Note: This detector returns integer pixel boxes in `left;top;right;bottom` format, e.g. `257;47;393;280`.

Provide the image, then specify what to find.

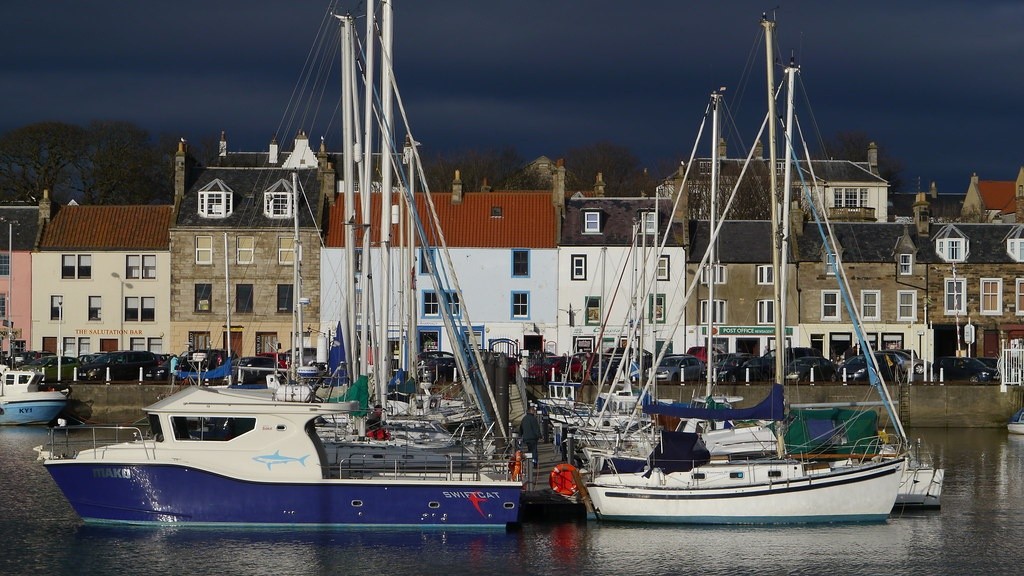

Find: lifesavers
509;456;527;474
549;463;579;497
877;431;889;444
512;451;521;480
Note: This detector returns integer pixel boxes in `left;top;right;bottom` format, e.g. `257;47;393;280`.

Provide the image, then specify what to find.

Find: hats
374;405;381;408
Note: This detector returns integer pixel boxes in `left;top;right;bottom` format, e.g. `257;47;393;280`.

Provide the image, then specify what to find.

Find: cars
14;348;316;385
416;351;458;382
933;356;1022;383
508;346;831;386
831;349;930;382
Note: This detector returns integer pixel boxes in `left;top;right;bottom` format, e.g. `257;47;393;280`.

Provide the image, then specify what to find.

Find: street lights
0;217;20;358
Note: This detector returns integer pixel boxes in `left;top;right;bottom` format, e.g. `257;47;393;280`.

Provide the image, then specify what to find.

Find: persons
585;352;592;368
519;406;543;469
370;405;382;421
560;353;566;373
170;355;179;380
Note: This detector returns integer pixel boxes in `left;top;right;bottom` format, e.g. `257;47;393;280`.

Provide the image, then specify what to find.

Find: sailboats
34;4;540;541
530;5;945;525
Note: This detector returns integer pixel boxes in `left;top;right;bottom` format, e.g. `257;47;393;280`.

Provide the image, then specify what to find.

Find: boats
0;363;69;425
1007;407;1024;434
